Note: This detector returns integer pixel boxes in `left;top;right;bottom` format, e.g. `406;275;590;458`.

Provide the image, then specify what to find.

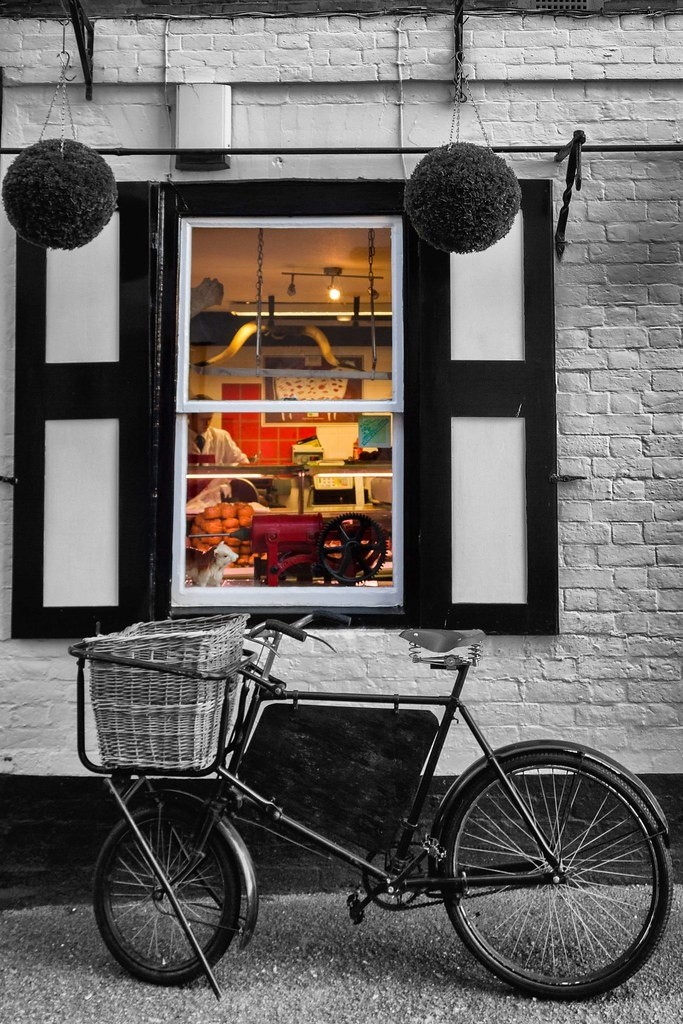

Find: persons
185;393;267;518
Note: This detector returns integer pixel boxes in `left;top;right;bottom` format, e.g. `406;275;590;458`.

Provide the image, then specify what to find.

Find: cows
185;541;239;588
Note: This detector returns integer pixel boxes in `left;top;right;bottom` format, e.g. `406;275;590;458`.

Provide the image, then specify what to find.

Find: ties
195;435;204;451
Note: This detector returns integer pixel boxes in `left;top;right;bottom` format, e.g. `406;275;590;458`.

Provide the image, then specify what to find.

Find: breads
186;500;267;568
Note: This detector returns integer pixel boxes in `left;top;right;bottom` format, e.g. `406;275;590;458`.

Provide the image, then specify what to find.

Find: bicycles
68;610;672;1000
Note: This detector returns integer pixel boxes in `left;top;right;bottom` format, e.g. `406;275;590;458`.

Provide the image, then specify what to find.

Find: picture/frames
259;354;364;427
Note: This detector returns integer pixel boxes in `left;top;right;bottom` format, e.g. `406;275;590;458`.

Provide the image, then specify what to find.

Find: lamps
278;265;384;303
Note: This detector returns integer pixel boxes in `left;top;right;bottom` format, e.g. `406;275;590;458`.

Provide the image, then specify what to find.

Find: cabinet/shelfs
186;460;393;588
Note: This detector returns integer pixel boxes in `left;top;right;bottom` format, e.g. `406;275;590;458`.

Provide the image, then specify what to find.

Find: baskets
84;612;252;770
